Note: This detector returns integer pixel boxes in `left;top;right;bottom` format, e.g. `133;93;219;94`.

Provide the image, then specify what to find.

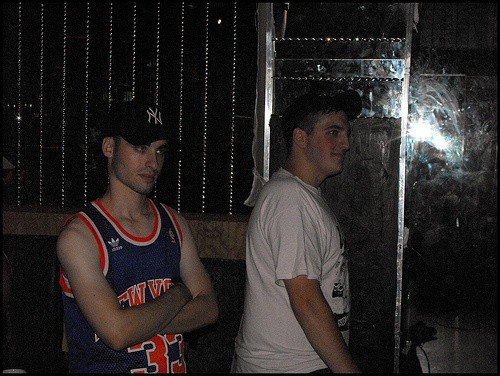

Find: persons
230;90;364;376
57;97;218;376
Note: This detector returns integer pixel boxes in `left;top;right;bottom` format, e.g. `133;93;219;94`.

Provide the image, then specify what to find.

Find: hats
280;88;363;144
103;98;173;146
